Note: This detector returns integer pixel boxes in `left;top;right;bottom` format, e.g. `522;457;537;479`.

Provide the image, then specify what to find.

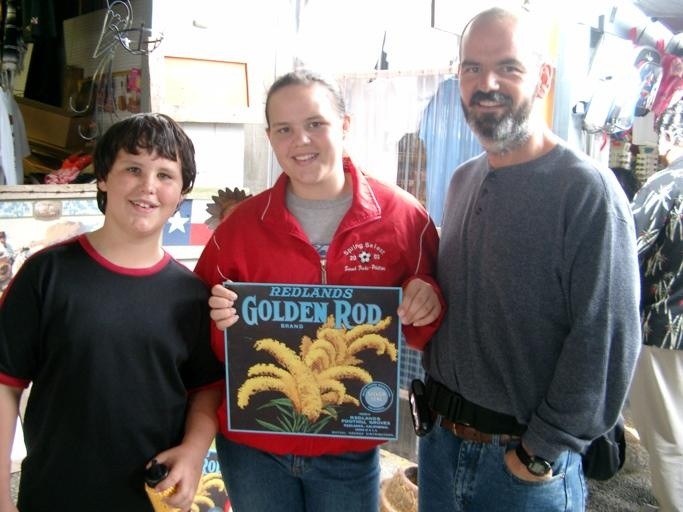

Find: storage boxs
10;95;89;151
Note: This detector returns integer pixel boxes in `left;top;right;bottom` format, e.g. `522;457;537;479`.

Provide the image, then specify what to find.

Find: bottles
143;464;183;512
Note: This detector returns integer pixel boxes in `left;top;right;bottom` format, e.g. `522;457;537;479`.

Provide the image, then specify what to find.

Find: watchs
516;439;552;477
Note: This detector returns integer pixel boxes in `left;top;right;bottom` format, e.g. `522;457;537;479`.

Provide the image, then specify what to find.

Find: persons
407;5;643;512
630;102;683;512
0;114;222;512
611;167;642;201
190;69;446;512
0;231;20;290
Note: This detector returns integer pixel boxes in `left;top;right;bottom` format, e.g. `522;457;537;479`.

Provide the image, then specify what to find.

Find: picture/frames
157;45;263;127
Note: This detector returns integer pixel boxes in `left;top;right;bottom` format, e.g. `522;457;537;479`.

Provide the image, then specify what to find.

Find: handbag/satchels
578;415;626;481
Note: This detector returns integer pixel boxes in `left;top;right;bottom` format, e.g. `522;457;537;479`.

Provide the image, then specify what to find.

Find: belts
440;417;519;446
